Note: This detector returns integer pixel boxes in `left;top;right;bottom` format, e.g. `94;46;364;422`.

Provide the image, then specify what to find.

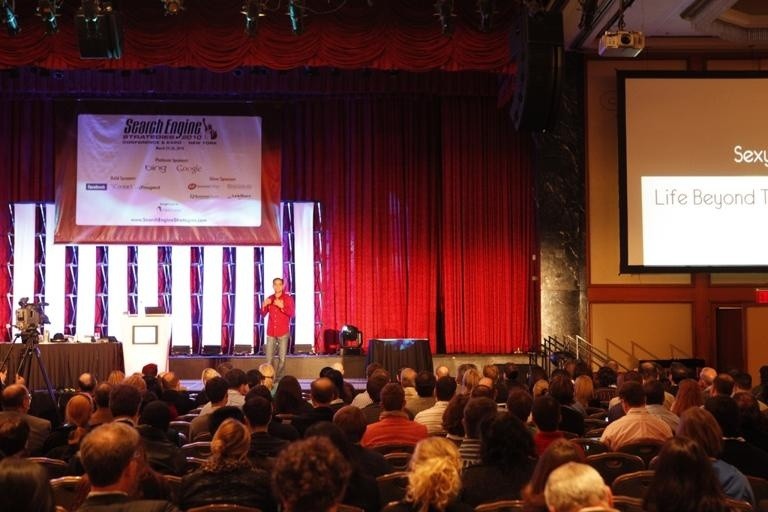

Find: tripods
0;332;58;409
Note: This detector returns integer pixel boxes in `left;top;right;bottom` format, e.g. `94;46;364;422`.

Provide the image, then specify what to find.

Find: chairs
0;376;768;512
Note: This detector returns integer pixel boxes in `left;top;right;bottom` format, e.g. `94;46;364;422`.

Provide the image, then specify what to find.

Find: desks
0;339;125;392
366;338;433;383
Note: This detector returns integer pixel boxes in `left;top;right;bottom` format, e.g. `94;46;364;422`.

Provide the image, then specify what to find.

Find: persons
1;379;53;453
0;361;27;404
0;459;57;511
260;278;295;382
47;361;768;512
0;415;29;456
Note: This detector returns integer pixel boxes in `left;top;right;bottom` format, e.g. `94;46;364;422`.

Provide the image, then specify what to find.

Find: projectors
599;31;645;57
